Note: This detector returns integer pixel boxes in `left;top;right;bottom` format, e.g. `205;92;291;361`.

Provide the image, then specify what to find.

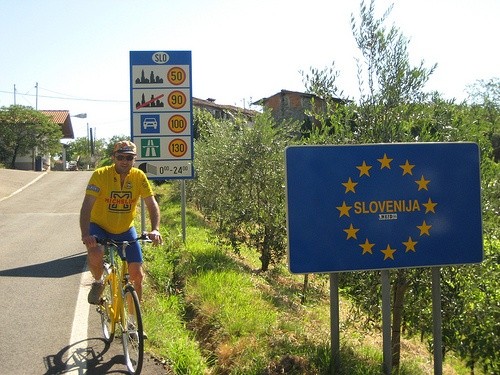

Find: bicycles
83;232;164;375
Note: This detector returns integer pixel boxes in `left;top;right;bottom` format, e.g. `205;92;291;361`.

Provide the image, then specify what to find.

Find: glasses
115;155;133;161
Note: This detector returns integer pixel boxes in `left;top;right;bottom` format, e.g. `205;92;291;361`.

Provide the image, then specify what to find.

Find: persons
79;141;164;339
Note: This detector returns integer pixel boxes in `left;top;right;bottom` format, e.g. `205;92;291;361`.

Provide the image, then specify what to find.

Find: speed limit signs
168;114;187;133
167;66;186;85
169;137;188;157
167;89;187;110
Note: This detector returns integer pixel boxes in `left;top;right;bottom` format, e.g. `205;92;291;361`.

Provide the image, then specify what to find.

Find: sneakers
128;321;148;340
88;275;107;305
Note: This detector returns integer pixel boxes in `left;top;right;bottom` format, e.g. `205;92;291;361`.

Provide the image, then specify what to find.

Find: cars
66;160;79;171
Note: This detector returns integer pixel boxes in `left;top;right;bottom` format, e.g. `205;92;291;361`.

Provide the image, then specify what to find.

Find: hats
112;141;137;155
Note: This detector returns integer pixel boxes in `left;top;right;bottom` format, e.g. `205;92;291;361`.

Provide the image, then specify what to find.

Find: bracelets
151;228;158;231
